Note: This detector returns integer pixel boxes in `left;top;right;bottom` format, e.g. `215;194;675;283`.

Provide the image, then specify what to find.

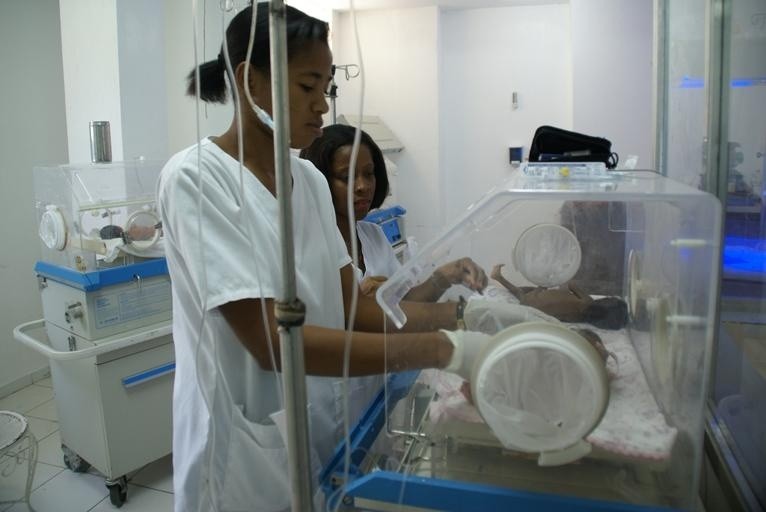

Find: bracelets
431;273;446;292
456;296;466;331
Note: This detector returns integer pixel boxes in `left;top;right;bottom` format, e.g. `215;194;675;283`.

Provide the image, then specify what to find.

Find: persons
459;329;607;404
300;124;489;427
490;263;629;331
100;223;163;240
158;2;561;512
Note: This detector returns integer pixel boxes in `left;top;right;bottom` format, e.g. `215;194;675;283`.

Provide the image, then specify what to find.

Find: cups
88;120;112;163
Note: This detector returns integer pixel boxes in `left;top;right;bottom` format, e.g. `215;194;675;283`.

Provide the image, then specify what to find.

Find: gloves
463;295;559;333
437;328;492;380
